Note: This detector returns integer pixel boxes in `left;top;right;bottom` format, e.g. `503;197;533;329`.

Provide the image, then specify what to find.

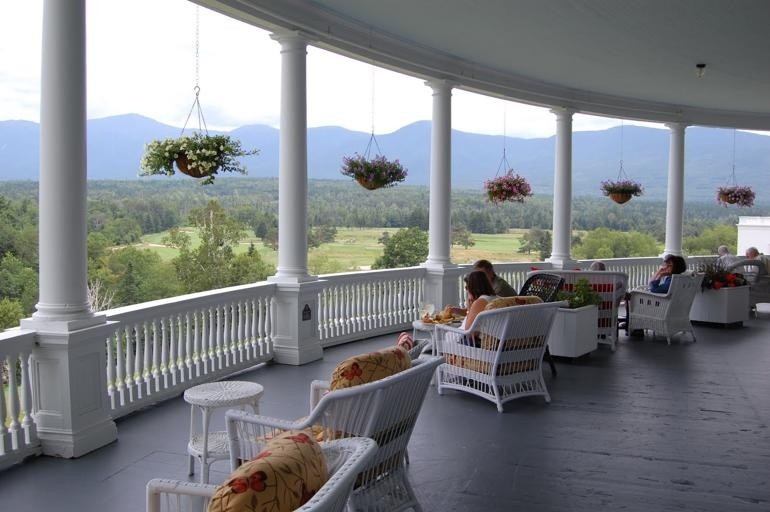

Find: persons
618;253;685;338
715;245;744;277
473;258;519;296
746;247;764;274
448;271;502;365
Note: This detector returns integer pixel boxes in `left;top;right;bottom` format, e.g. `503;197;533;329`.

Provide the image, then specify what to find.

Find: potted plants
689;262;751;328
539;277;604;367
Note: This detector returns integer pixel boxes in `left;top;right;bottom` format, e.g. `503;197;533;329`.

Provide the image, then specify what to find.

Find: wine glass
419;303;434;321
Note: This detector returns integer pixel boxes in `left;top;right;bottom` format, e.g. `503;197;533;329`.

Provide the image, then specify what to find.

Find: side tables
411;317;464;386
183;380;265;484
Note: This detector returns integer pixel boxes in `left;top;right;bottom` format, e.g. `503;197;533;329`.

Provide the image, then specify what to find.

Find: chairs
526;270;629;352
144;438;378;512
436;298;571;413
225;355;445;512
628;269;706;345
727;258;770;321
518;272;565;376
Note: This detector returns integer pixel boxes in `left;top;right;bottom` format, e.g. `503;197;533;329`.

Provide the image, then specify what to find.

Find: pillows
329;347;411;493
207;430;329;512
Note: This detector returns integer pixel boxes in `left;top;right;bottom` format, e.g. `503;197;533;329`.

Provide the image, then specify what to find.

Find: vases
721;193;746;204
493;189;514;198
356;175;387;190
609;191;634;204
174;153;220;178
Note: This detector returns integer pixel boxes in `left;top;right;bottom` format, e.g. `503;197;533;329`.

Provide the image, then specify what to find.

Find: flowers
599;177;646;198
713;184;756;209
136;132;261;185
339;151;409;189
483;166;535;206
